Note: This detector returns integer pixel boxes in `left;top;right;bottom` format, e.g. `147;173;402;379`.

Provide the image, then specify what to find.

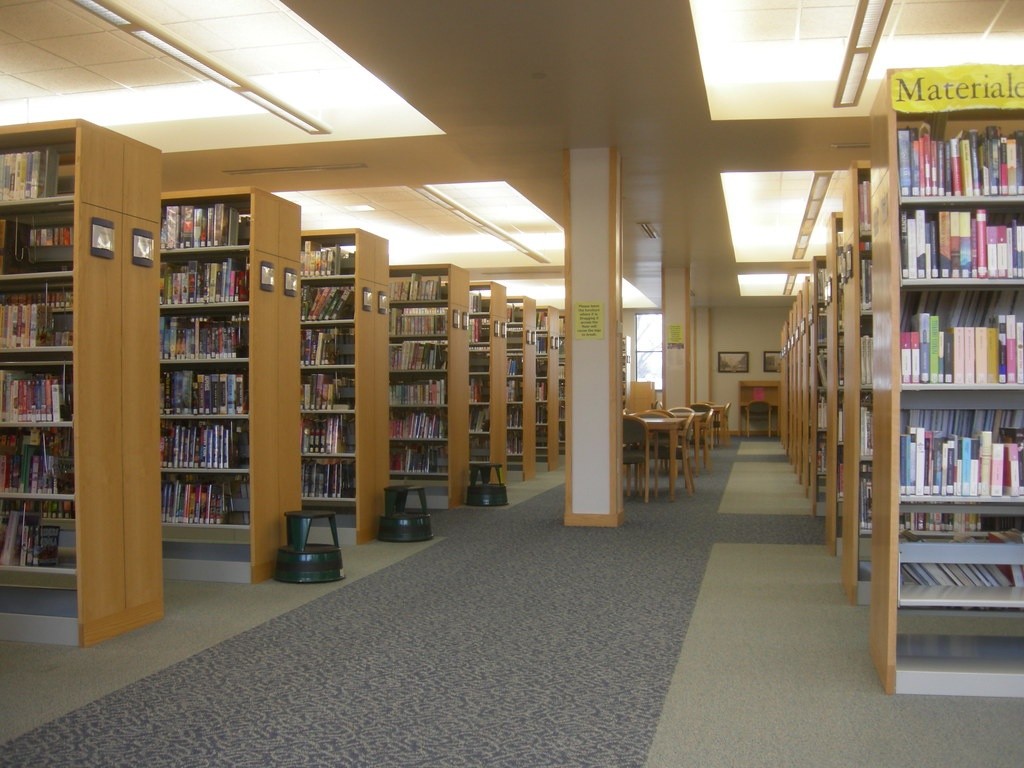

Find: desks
739;380;781;438
639;418;689;500
673;411;708;479
710;404;726;447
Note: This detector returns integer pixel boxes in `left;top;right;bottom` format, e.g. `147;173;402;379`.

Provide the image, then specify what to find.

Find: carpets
0;434;829;768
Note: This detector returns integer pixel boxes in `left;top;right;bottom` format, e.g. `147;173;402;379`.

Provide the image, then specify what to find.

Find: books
836;232;844;511
508;306;523;459
859;182;875;537
301;238;354;501
1;150;74;580
534;310;548;460
557;337;567;457
468;293;490;461
161;204;249;528
897;128;1024;607
389;271;448;480
816;269;827;473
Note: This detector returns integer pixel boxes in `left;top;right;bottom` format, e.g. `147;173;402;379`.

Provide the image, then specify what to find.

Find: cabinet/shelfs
630;382;656;410
506;295;538;482
717;351;749;374
389;263;472;511
536;305;560;471
299;228;390;545
779;69;1024;696
160;188;306;589
557;308;564;456
468;281;509;488
623;334;631;412
1;119;163;650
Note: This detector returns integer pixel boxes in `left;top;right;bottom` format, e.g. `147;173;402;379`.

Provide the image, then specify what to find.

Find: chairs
745;400;772;439
623;400;732;503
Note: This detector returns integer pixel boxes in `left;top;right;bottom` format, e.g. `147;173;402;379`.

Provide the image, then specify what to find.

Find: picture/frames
763;350;782;373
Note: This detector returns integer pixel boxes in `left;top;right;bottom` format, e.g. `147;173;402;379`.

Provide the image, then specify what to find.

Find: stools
273;508;342;584
377;484;433;542
465;460;509;507
